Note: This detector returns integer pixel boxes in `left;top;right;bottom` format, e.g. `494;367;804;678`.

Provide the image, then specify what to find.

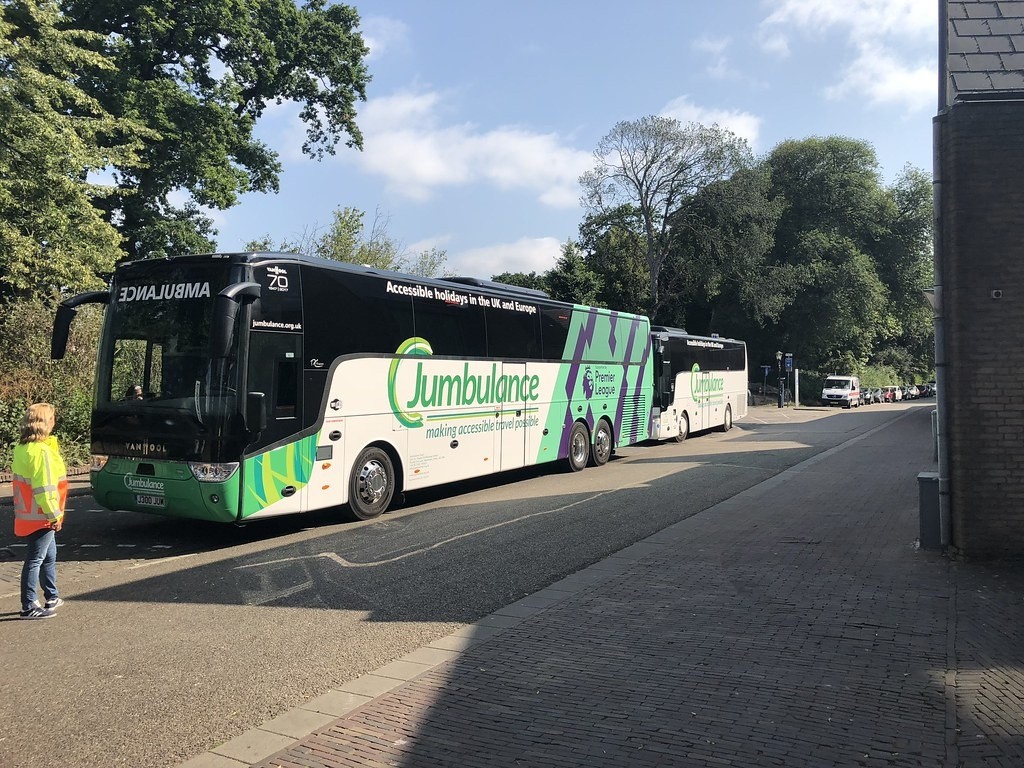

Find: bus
51;248;654;526
650;326;749;443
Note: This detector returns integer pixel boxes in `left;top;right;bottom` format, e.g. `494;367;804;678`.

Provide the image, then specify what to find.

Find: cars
885;386;903;401
907;386;920;399
880;387;895;402
860;387;874;405
926;385;934;396
870;388;884;403
899;386;912;400
916;385;929;397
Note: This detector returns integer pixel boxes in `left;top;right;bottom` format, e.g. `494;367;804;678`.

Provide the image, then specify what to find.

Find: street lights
775;351;783;408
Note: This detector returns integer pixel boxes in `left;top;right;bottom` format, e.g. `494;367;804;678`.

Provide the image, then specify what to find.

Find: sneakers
45;598;64;612
19;602;58;619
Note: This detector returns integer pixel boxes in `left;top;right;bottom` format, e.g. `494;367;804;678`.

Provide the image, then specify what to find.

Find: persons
13;403;65;619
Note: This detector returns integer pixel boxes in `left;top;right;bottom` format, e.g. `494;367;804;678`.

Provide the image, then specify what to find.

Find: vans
821;376;859;407
